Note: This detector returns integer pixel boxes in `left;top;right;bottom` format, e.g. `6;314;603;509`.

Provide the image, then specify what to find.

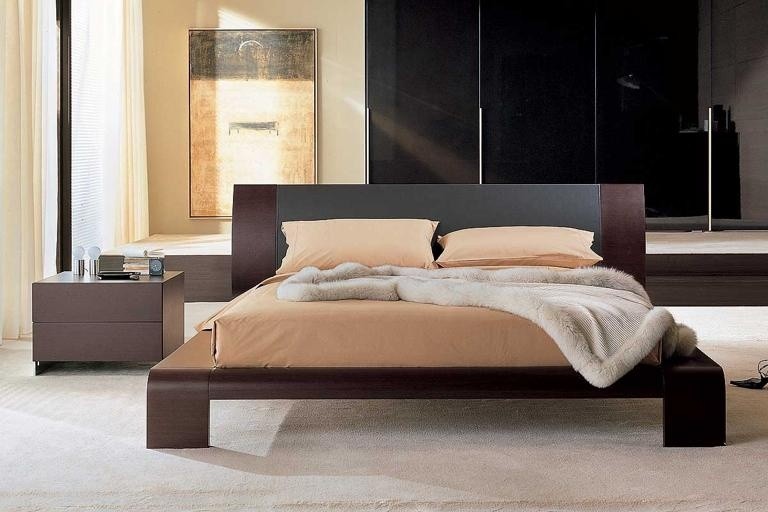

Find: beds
146;184;725;449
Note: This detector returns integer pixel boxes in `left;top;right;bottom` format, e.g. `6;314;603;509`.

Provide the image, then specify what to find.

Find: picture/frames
188;27;318;220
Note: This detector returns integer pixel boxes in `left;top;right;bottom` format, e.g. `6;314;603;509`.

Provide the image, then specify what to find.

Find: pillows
433;225;603;270
275;219;441;275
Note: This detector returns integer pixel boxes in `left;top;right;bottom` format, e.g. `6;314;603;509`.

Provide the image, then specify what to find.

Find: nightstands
32;269;184;376
